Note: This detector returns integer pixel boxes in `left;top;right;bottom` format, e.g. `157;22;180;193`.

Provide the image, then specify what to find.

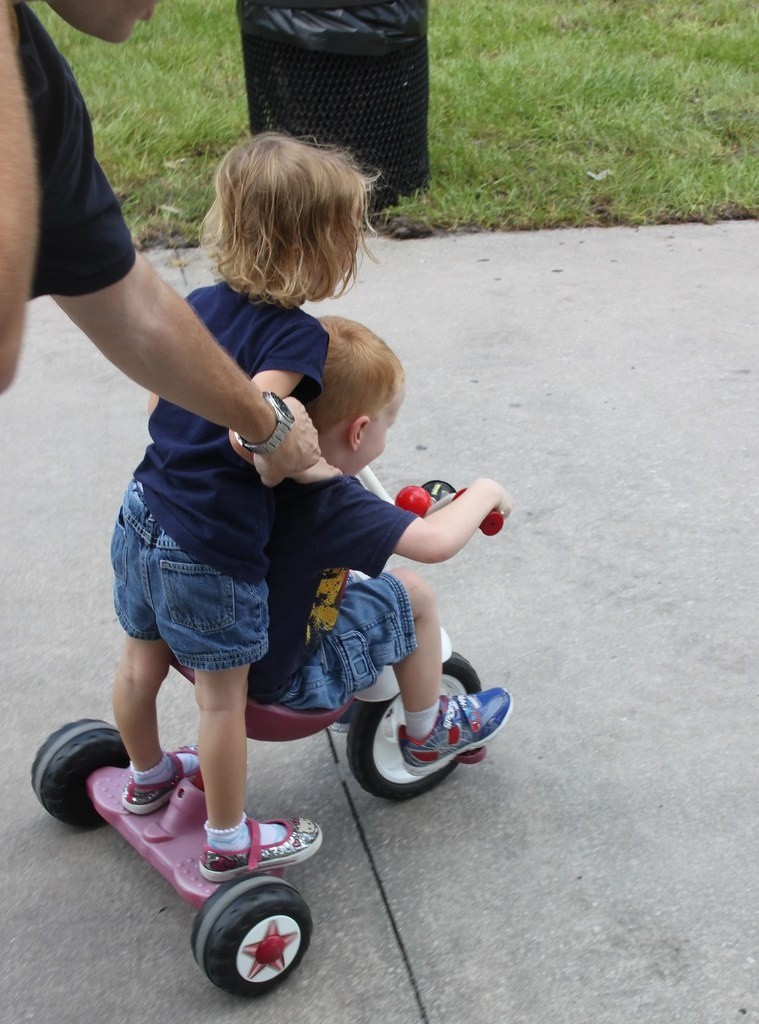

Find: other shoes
199;818;322;882
122;743;201;815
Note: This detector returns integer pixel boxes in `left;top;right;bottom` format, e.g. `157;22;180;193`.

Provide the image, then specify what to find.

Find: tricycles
34;480;503;998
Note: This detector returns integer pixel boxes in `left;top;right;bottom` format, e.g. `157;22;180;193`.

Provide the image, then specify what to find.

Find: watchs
232;391;295;457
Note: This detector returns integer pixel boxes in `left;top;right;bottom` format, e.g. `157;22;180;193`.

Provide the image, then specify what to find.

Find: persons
109;122;515;884
0;0;323;487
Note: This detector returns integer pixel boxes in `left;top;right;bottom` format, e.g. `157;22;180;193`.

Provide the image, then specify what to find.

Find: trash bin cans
234;0;430;222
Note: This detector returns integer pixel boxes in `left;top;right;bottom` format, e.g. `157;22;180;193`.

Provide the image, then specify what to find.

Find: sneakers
398;687;512;776
